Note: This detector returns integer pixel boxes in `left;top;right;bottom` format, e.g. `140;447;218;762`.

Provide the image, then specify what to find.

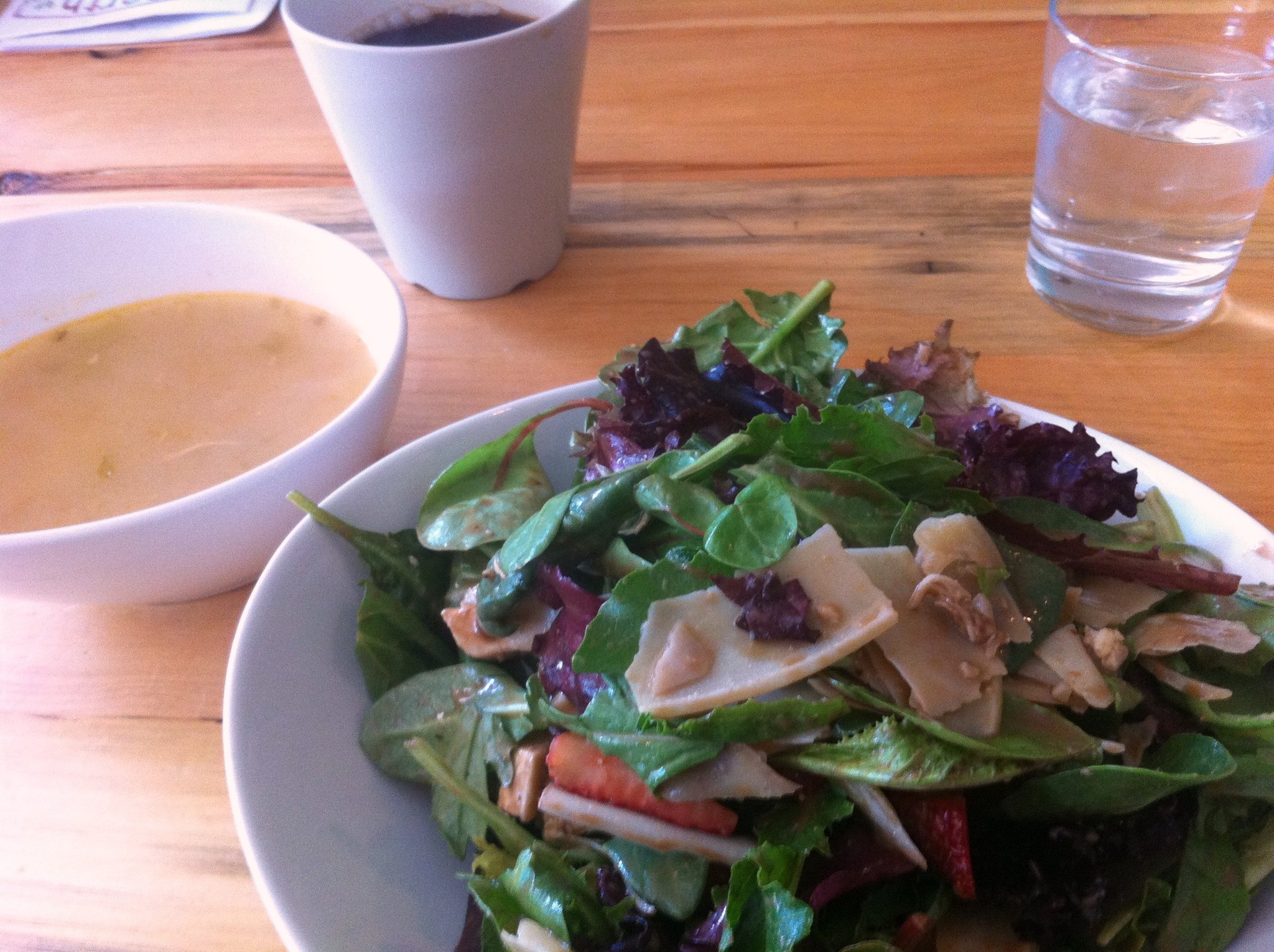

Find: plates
220;379;1274;952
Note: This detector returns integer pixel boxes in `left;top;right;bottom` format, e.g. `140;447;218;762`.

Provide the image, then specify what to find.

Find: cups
1026;0;1273;339
279;1;592;300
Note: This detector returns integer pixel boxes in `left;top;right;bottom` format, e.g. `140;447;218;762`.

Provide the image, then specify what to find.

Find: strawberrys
901;784;975;898
545;731;738;836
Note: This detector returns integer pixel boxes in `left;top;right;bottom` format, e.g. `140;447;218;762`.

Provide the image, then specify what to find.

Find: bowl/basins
0;203;406;605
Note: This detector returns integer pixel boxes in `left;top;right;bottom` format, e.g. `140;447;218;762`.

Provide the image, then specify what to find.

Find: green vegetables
285;278;1273;952
